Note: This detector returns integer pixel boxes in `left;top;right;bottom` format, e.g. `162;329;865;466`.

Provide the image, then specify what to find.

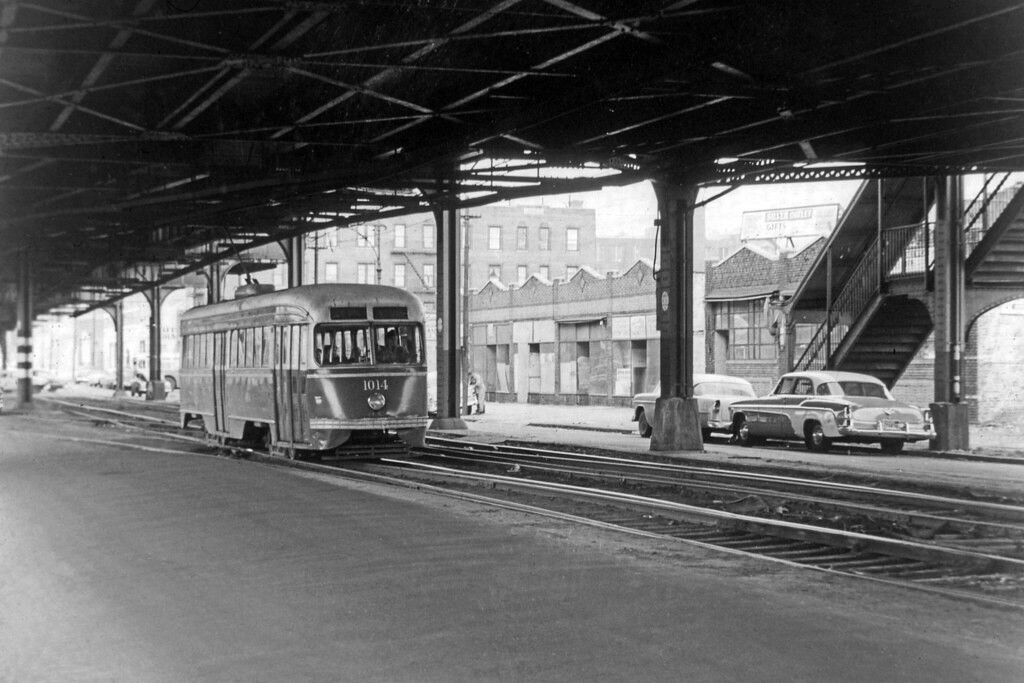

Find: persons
468;371;486;414
379;330;411;364
314;348;321;365
349;347;362;363
332;346;340;364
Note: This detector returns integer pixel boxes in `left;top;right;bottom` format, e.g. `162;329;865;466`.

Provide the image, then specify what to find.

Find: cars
728;371;937;455
163;364;181;391
632;374;758;441
128;371;172;398
74;366;133;390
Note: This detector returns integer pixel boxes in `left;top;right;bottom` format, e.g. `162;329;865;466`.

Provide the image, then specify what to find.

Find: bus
179;283;447;460
